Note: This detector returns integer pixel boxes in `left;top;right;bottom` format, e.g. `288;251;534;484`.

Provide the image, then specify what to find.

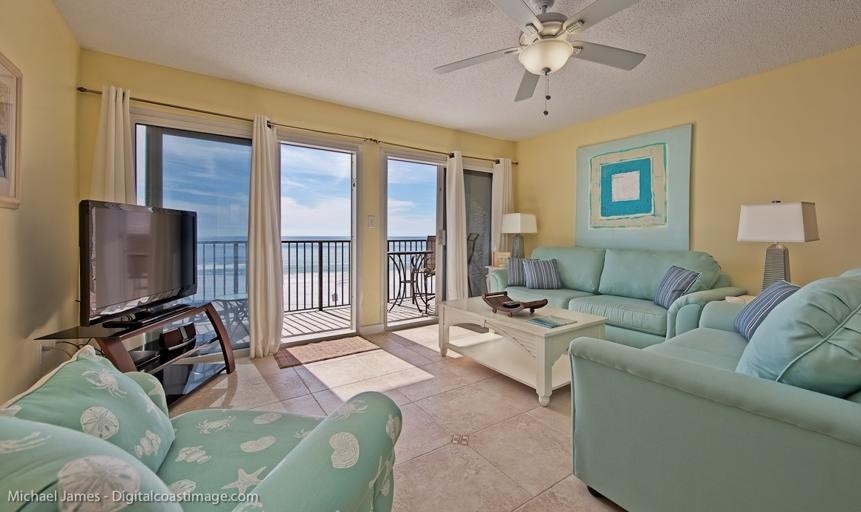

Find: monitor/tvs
78;198;197;327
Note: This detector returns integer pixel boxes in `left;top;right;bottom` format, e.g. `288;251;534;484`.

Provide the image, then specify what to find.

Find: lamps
735;200;821;295
500;210;539;257
515;38;575;115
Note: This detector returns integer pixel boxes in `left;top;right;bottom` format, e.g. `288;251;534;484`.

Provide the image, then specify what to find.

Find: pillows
507;256;563;288
4;341;179;471
655;263;704;308
735;269;861;398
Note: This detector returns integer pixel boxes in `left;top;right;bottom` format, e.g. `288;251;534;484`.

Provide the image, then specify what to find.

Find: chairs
0;368;409;509
564;269;861;512
413;234;437;311
465;230;480;296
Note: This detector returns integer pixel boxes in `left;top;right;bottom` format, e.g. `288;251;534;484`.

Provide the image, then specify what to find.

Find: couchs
487;244;732;349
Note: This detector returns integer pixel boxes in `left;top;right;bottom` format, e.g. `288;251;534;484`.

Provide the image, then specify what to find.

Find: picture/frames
1;53;24;212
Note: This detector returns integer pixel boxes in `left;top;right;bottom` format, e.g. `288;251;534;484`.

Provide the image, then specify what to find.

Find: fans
434;1;650;103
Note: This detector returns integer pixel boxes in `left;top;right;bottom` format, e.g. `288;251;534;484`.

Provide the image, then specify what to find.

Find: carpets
273;335;384;368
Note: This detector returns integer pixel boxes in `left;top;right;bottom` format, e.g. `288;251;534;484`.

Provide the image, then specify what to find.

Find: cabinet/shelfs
32;297;239;409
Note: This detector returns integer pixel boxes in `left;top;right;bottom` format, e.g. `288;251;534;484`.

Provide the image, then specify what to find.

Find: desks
386;250;435;315
216;293;250;344
